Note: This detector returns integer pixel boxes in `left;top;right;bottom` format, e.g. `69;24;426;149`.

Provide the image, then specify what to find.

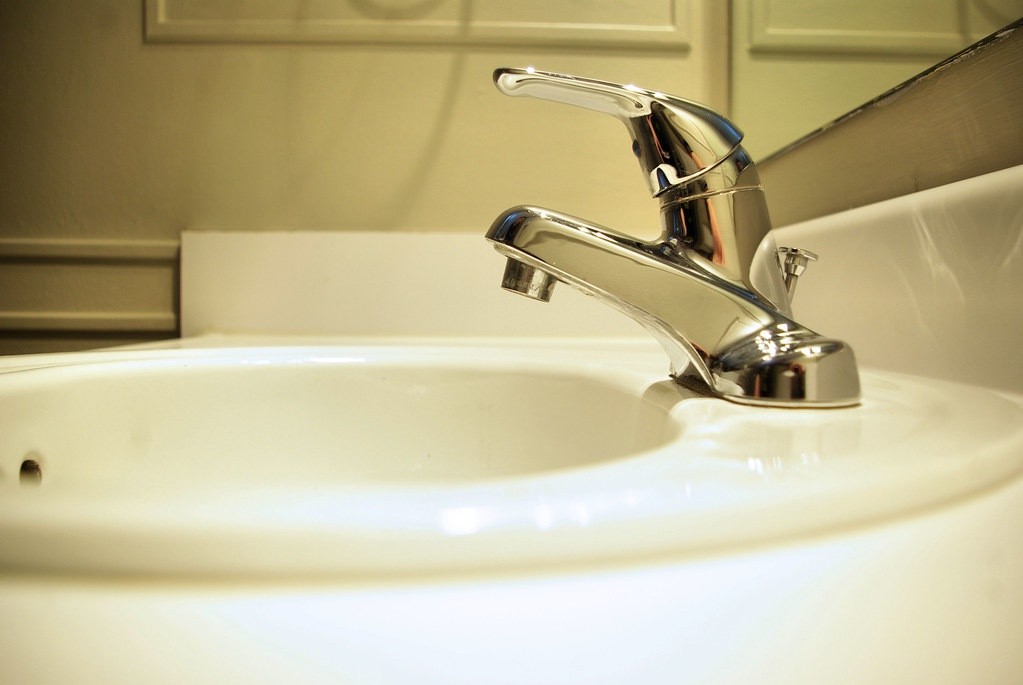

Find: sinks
0;339;1022;663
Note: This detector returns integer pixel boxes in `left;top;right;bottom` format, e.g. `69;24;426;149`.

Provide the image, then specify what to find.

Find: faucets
483;66;863;411
